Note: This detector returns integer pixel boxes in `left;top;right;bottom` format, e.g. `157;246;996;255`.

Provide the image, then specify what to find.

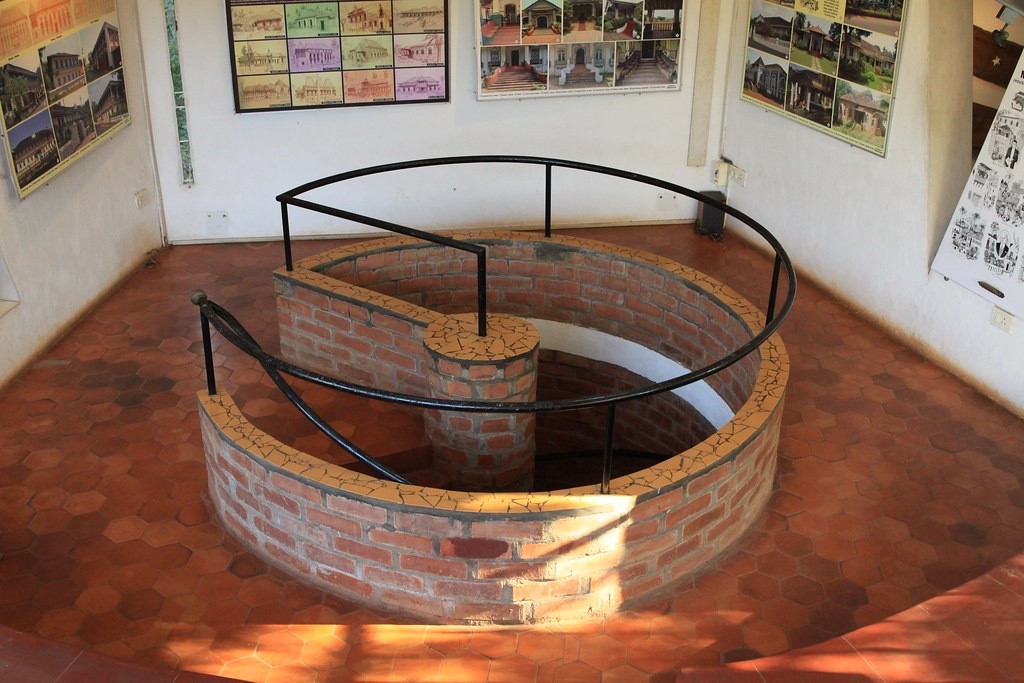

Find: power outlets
134;189;149;210
728;164;746;187
